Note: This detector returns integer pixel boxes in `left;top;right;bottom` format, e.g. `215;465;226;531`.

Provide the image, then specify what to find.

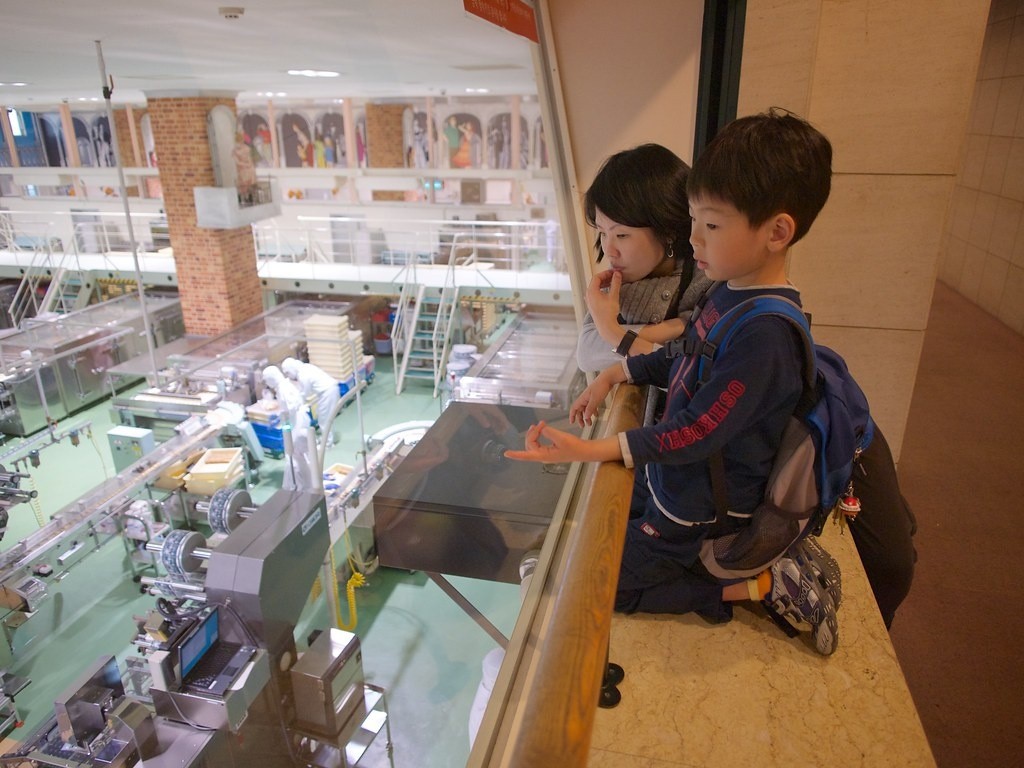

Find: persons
576;141;918;634
501;105;866;659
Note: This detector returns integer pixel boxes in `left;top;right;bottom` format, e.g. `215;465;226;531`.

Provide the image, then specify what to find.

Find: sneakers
765;539;841;660
783;529;841;615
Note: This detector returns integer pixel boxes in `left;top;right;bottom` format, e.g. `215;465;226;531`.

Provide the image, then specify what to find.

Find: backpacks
663;274;876;583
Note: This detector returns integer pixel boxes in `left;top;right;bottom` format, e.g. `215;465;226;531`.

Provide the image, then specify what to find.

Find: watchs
608;328;639;361
